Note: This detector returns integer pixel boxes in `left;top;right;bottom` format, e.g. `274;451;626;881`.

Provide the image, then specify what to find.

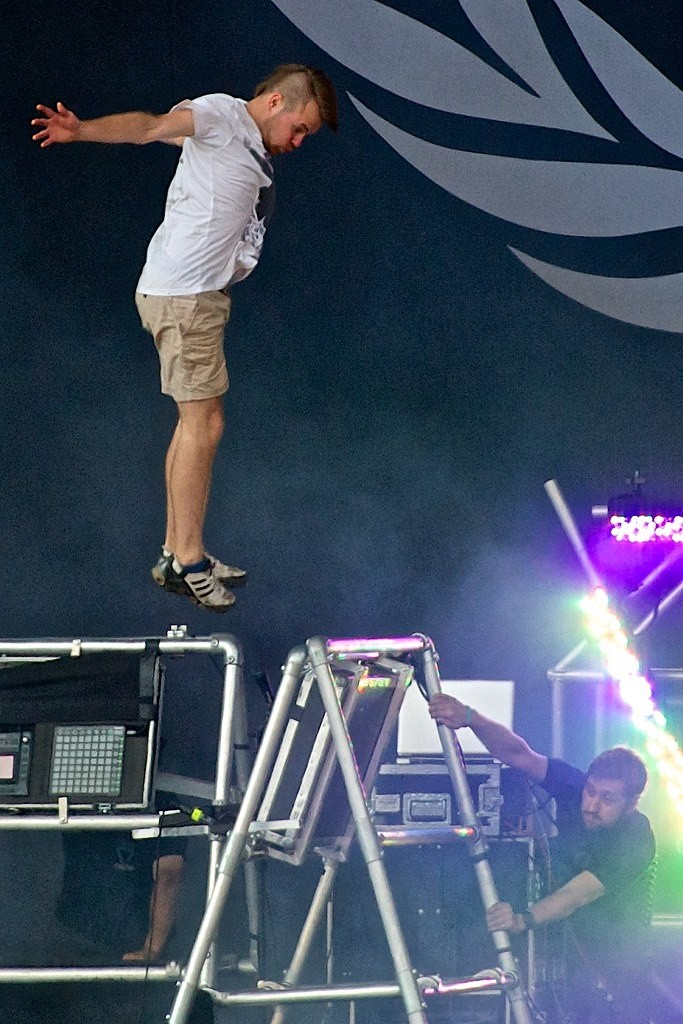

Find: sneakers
156;547;248;591
152;554;236;613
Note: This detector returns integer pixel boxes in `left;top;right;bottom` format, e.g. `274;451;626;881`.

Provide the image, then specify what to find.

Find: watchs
518;910;532;934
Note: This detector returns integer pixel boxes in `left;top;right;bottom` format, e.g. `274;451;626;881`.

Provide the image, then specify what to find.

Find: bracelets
465;705;471;728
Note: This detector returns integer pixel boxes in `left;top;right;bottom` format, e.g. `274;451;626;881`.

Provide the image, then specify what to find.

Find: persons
429;689;659;1024
48;772;192;1024
29;63;334;613
